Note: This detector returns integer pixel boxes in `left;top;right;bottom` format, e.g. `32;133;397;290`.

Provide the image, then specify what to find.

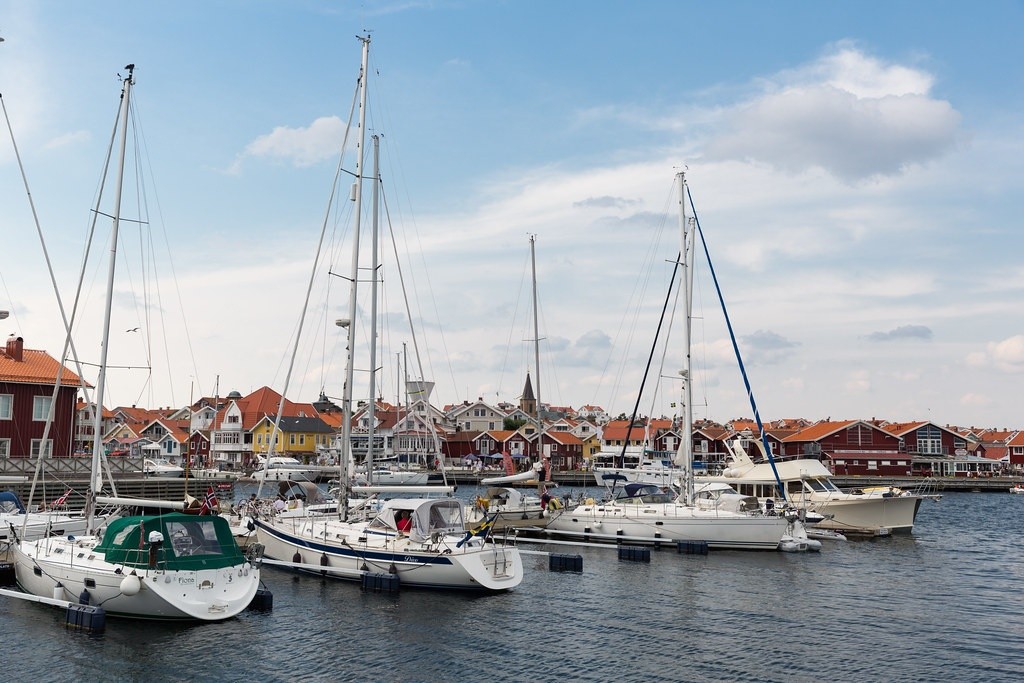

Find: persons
289;495;299;511
396;511;412;531
921;466;926;476
247;493;263;518
270;496;284;517
169;458;178;465
434;458;438;471
244;456;248;468
229;503;242;519
161;456;168;464
465;459;477;470
928;467;932;477
888;485;911;497
181;457;187;464
533;465;560;510
254;457;259;468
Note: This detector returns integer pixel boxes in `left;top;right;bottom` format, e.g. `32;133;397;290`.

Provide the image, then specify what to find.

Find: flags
198;486;219;517
50;487;73;509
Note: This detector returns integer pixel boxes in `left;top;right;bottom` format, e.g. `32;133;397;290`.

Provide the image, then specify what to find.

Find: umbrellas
464;453;478;460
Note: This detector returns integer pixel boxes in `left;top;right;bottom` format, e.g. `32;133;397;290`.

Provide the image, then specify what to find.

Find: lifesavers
548;499;560;512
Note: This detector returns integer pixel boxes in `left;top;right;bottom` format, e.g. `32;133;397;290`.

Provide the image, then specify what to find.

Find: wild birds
126;327;141;333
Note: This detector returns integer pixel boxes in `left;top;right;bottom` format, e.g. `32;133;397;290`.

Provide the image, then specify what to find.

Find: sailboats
0;34;925;622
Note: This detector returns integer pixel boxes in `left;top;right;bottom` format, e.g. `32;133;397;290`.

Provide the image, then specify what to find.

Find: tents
477;452;526;472
